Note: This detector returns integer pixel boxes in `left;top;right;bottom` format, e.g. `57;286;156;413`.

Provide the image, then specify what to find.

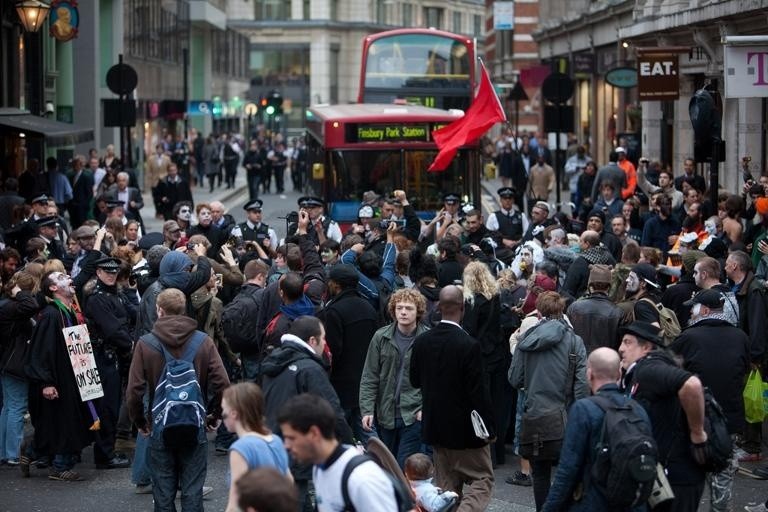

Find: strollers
355;434;464;512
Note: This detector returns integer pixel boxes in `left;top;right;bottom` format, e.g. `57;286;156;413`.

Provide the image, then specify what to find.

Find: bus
357;26;485;120
305;100;486;227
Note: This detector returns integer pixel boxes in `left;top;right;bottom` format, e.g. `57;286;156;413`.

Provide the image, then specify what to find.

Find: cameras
747;179;757;187
129;267;142;287
286;211;304;221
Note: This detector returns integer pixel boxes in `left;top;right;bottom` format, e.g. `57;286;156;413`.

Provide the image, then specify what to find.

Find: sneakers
731;432;768;511
134;481;153;496
0;450;81;481
505;471;532;488
175;483;213;500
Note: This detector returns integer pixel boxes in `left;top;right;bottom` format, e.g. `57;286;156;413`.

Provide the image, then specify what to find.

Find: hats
682;288;725;308
535;204;548;211
103;200;125;213
443;194;462;205
326;265;359;288
587;208;605;225
497;187;516;198
587;263;613;297
632;262;659;288
77;224;95;238
29;189;60;226
620;322;661;345
297;195;326;208
407;250;438;281
521;274;555;314
139;220;181;252
243;198;263;212
616;146;627;153
92;255;123;274
358;188;407;236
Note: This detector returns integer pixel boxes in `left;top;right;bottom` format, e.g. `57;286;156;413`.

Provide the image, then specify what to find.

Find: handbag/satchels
470;410;489;439
517;409;568;463
632;299;681;347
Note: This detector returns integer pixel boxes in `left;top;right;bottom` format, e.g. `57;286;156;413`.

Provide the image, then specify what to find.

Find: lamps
11;0;50;41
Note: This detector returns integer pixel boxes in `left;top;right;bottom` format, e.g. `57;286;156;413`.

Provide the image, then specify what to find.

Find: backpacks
220;287;260;355
378;281;393;327
633;356;734;475
140;332;209;461
584;395;659;512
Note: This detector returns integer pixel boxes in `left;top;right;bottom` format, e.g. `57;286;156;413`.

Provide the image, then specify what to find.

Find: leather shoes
95;439;136;470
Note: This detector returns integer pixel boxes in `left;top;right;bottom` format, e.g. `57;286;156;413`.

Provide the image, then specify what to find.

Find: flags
427;66;505;171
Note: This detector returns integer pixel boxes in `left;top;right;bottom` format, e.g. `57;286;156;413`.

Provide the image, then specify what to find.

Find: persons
0;125;766;512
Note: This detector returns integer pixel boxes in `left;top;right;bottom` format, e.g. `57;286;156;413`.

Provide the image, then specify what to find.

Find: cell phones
642;160;650;169
187;243;198;251
743;157;751;161
603;206;608;212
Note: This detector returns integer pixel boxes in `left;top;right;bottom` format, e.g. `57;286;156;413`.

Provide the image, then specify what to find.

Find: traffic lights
257;98;275;120
274;95;287;115
